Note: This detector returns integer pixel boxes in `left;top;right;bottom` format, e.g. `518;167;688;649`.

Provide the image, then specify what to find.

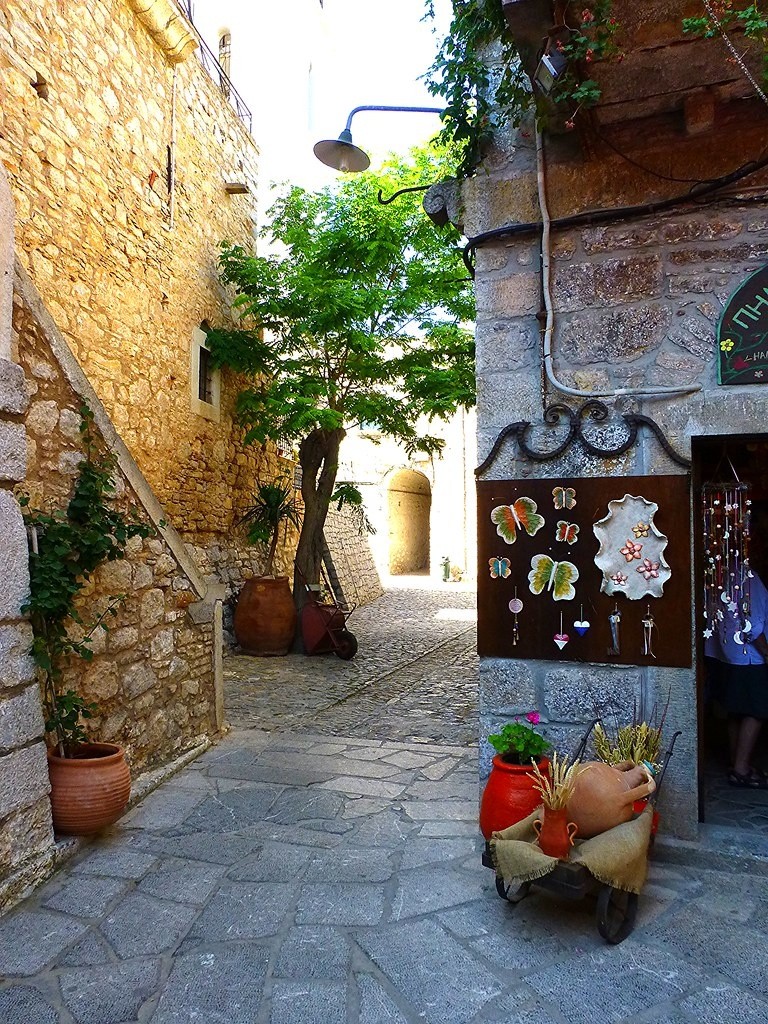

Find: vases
532;803;579;859
480;756;555;842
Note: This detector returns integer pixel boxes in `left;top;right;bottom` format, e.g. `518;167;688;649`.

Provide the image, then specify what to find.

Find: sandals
727;764;764;777
729;769;768;789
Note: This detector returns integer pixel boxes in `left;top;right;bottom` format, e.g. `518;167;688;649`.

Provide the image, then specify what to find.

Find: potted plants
11;397;168;832
232;475;303;656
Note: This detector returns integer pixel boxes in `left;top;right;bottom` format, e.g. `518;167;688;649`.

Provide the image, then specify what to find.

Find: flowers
488;710;549;766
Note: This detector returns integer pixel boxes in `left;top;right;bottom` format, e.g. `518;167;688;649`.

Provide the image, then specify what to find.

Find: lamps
311;106;447;175
532;38;566;97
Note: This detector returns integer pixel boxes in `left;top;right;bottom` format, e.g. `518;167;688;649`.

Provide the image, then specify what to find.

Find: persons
702;529;768;785
